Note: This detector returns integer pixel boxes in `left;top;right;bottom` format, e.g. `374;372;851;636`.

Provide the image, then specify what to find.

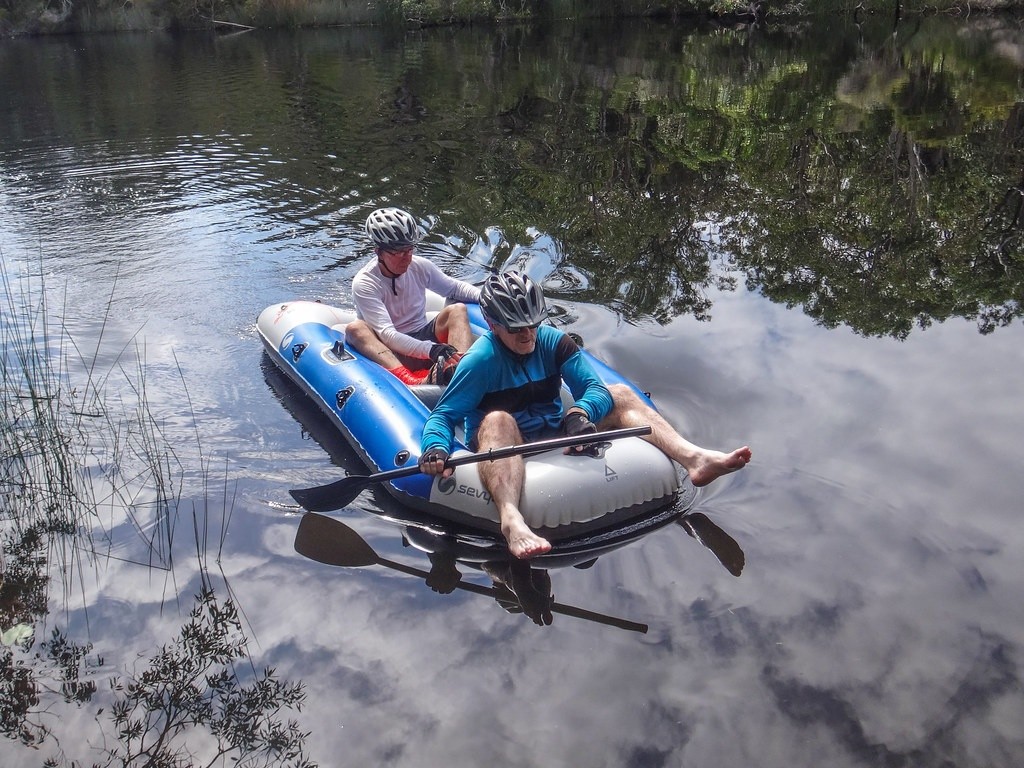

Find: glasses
497;321;541;334
381;246;418;258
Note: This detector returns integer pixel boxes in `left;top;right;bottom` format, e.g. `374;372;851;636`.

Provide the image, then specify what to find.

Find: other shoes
443;364;457;386
417;355;446;385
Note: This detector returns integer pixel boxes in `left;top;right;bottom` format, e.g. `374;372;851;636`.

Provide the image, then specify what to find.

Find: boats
251;298;682;547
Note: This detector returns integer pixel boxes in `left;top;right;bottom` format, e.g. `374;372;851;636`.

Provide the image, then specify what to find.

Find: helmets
365;206;419;250
479;269;549;328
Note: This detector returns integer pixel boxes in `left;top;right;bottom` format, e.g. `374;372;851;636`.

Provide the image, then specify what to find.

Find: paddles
287;426;654;512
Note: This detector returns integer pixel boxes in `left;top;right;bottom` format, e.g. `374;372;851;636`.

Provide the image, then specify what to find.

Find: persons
344;207;484;386
397;511;745;627
420;271;752;561
